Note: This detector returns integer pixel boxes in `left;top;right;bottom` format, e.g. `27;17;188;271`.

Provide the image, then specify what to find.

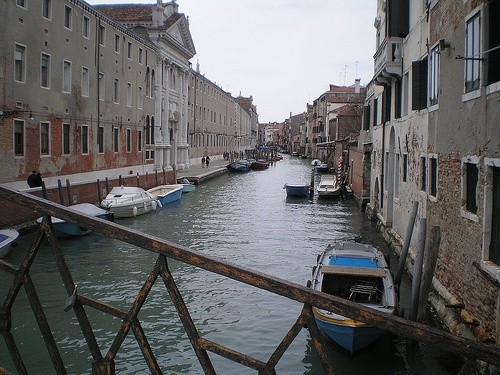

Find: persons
202;156;205;168
206;156;209;168
223;152;229;161
27;170;43;187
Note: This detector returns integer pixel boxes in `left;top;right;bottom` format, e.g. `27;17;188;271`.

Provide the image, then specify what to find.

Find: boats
100;187;157;218
316;174;341;197
228;158;269;173
311;158;328;173
282;183;311;197
268;149;307;161
37;202;115;237
307;234;396;358
145;176;196;206
0;228;20;259
